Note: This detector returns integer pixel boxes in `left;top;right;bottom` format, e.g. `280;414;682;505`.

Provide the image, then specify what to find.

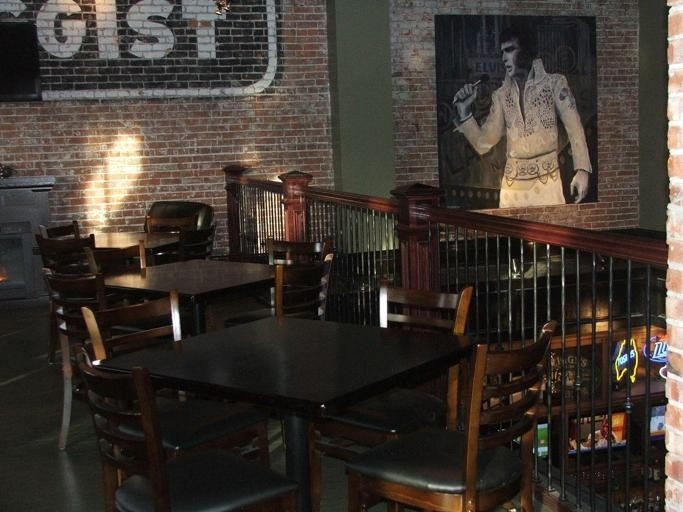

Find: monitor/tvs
568;409;628;454
0;19;43;102
513;423;551;461
648;402;667;444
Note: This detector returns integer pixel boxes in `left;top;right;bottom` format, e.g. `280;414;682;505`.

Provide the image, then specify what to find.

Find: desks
2;175;57;310
37;200;347;418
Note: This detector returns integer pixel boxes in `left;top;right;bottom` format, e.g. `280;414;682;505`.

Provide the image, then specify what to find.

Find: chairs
346;319;552;510
311;281;476;510
76;348;272;511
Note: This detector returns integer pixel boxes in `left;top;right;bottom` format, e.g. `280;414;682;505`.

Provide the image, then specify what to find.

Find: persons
448;16;594;211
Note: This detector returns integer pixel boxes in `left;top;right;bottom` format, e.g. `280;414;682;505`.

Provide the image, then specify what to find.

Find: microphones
452;75;489;103
